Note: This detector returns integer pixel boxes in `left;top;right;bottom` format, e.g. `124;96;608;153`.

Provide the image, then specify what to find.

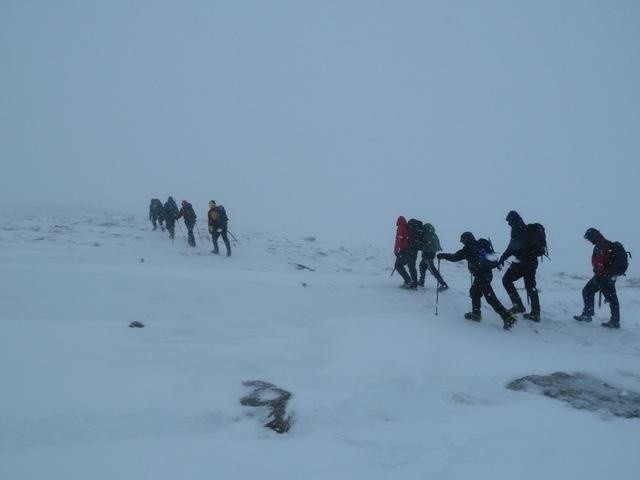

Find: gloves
497;257;504;271
437;253;447;259
395;252;399;256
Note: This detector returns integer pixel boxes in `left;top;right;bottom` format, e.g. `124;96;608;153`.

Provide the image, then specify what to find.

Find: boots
523;305;540;322
464;310;481;322
508;299;526;314
501;312;516;329
438;282;448;291
417;278;424;287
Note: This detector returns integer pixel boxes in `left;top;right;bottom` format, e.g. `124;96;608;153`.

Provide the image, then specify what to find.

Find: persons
149;198;165;232
572;227;621;328
393;215;418;289
416;223;448;291
497;210;541;322
437;232;515;329
176;200;197;247
207;200;231;257
160;197;179;239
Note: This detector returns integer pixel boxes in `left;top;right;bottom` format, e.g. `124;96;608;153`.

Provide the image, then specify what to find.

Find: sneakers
212;249;219;255
573;313;592;322
227;249;231;256
402;280;417;289
601;321;620;328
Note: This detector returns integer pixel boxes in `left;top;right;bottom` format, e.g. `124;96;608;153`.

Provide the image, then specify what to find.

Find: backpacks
215;205;228;224
607;242;628;275
525;223;546;258
473;238;499;271
422;223;440;252
407;219;423;250
